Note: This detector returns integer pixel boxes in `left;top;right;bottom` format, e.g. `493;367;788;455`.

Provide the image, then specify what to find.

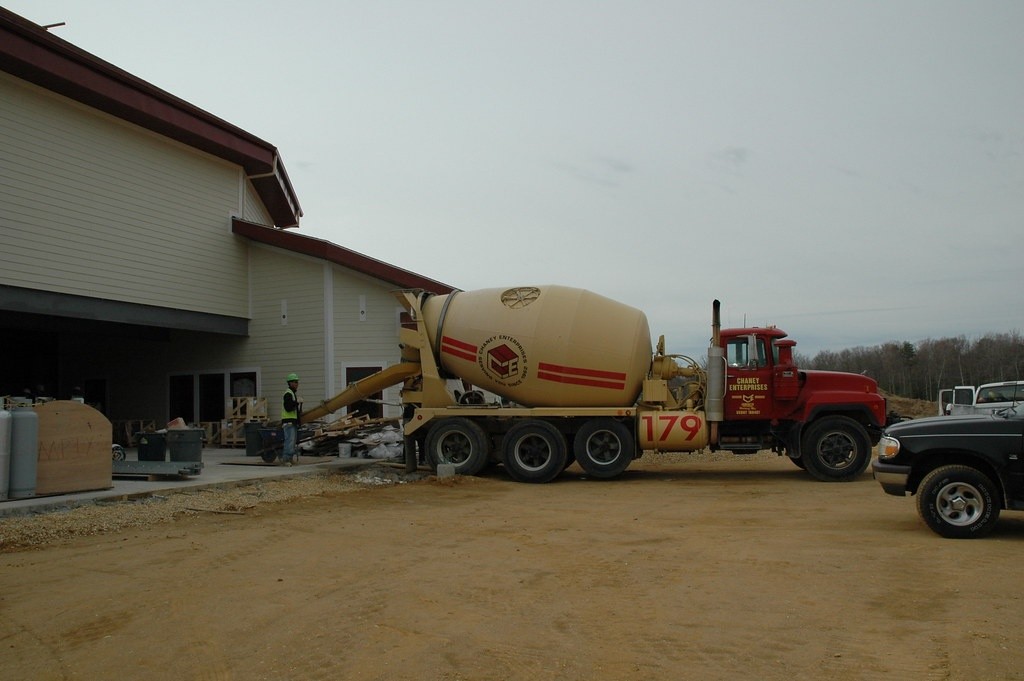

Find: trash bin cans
243;422;264;457
135;430;168;462
168;428;206;476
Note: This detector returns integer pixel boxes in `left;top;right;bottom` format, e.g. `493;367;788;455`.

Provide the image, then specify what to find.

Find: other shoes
283;460;295;467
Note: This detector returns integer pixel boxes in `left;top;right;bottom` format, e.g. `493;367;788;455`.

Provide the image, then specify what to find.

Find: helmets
286;373;299;381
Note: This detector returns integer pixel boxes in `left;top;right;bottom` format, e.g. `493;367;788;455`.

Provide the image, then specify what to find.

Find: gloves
297;396;303;403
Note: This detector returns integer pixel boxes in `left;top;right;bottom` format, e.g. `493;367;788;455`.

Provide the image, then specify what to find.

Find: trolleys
256;427;328;462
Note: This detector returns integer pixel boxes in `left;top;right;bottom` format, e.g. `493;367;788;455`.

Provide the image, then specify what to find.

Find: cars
872;381;1023;539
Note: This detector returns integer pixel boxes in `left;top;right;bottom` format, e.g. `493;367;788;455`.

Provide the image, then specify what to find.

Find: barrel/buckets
338;443;352;458
244;423;264;456
134;432;166;461
167;428;204;474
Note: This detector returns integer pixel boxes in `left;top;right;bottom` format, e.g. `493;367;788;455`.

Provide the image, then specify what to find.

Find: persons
281;373;303;467
989;391;1003;401
403;405;426;466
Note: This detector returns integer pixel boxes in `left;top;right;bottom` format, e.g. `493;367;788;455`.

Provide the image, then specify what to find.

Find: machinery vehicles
299;283;887;484
